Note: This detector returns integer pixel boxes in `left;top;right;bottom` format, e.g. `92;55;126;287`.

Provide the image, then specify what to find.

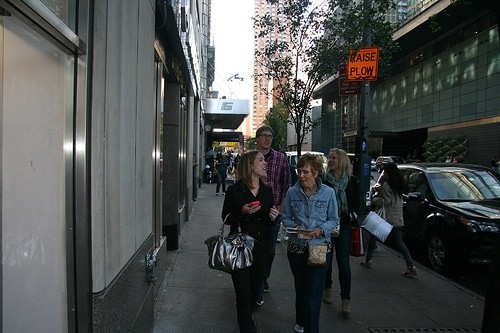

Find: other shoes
257;298;265;305
264;286;271;292
295;325;304;333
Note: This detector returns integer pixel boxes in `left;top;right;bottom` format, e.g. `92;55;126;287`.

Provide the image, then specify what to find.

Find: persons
320;149;362;316
280;154;339;333
222;151;281;333
444;154;461;164
360;162;420;278
490;158;500;175
273;150;297;244
247;125;291;307
203;146;243;196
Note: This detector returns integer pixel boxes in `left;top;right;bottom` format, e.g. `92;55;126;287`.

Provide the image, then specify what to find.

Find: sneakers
360;257;375;267
277;232;283;242
324;288;333;303
284;230;290;241
402;265;417;277
341;300;351;314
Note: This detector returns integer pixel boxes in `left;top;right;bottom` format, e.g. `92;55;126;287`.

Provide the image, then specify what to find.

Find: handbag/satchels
308;243;328;266
362;203;394;242
204;213;257;272
349;212;363;257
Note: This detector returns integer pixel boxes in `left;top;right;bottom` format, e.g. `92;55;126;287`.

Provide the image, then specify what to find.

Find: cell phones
250;201;260;208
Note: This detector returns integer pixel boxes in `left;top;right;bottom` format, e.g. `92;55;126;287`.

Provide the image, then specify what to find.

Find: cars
375;162;500;275
281;148;421;216
